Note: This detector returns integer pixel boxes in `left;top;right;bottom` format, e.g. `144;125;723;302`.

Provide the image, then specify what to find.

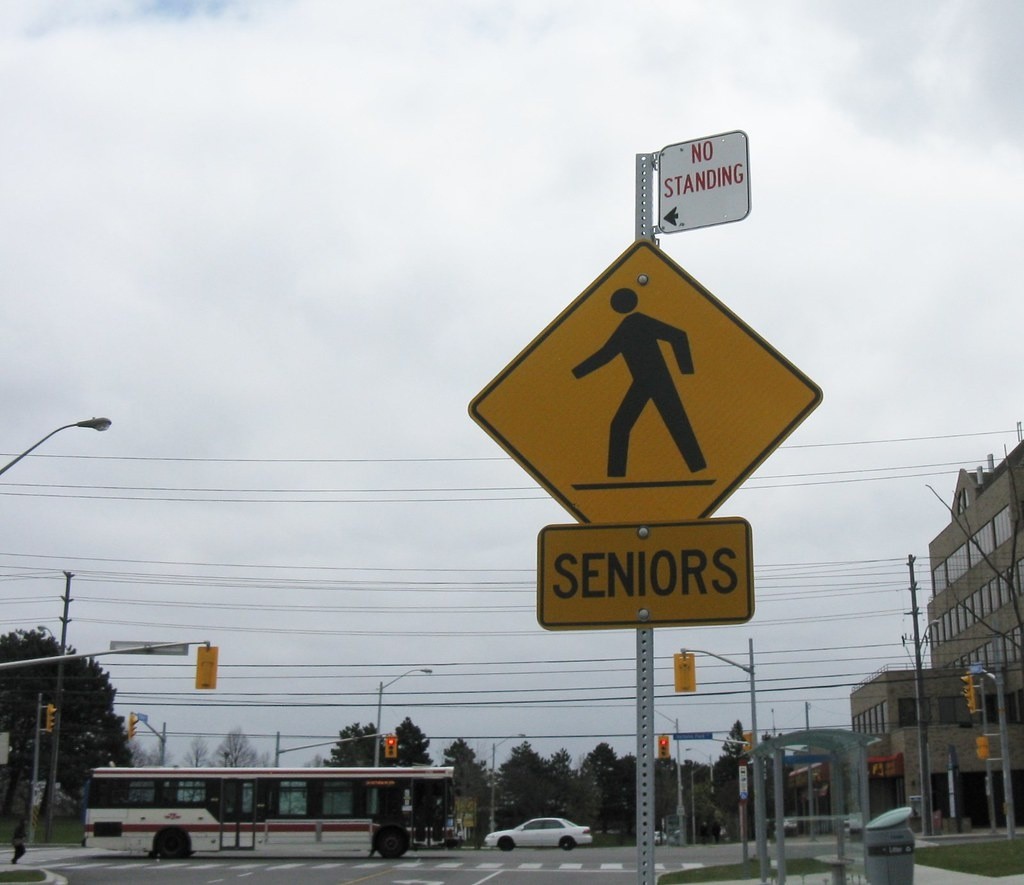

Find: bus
81;767;465;859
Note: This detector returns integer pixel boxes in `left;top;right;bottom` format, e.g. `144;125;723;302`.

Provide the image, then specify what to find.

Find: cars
484;818;592;851
782;819;797;828
645;831;667;844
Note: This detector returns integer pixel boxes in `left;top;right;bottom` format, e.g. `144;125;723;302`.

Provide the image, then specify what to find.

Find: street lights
489;733;526;850
686;747;714;793
913;621;942;837
375;669;432;768
37;625;65;843
0;417;112;477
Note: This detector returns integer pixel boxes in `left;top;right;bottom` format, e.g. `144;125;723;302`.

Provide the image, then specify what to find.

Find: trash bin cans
865;806;915;885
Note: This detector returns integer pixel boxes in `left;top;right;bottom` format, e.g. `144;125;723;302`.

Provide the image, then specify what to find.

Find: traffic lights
386;735;397;758
960;671;975;714
129;715;139;740
47;704;58;733
658;735;669;758
